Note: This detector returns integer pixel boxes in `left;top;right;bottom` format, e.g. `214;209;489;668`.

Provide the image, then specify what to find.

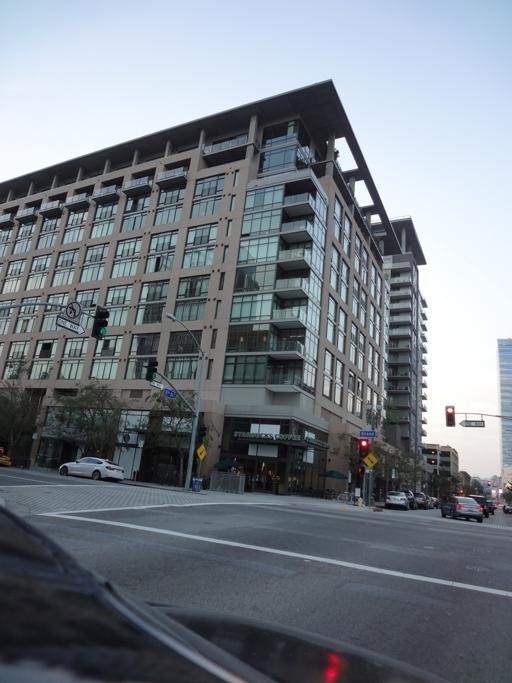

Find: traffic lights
445;405;455;426
358;466;365;473
146;360;159;382
359;438;369;457
91;305;110;340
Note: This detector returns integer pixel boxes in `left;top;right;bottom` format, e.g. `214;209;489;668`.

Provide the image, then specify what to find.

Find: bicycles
336;490;354;503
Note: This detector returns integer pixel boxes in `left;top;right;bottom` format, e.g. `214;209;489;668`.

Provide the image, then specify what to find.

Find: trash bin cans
192;477;203;492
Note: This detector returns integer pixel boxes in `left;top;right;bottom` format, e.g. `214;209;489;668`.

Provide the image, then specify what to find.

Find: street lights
166;312;209;489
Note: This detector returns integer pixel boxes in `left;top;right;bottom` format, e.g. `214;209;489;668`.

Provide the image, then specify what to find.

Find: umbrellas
212;456;240;471
318;469;348;488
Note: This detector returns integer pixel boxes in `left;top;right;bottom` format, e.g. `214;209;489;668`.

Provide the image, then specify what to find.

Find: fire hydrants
357;497;363;510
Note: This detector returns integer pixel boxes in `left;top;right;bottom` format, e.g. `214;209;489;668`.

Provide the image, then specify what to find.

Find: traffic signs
56;314;86;334
164;388;177;398
359;430;375;437
150;379;166;390
459;419;484;427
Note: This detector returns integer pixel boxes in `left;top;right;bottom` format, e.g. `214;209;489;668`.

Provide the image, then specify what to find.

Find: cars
1;453;12;466
58;456;126;483
385;486;511;524
